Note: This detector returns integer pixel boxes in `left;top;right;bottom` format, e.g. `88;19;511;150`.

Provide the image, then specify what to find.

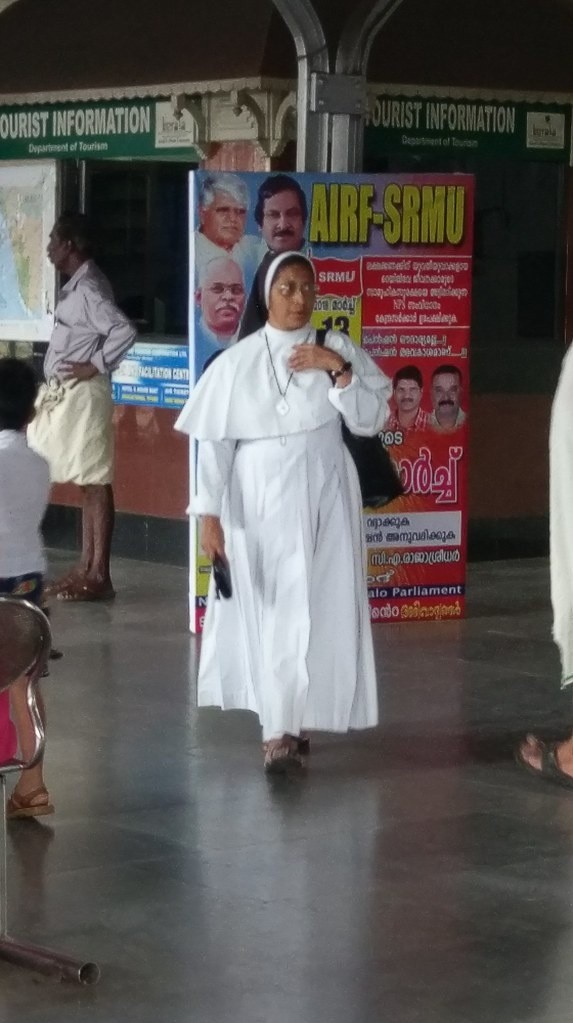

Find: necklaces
263;330;311;413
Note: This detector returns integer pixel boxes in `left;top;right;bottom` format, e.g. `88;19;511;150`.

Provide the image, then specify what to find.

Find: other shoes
259;734;303;787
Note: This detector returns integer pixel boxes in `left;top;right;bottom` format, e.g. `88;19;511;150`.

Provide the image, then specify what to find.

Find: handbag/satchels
316;328;403;507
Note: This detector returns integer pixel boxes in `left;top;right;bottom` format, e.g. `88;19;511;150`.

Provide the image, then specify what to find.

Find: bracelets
331;360;353;376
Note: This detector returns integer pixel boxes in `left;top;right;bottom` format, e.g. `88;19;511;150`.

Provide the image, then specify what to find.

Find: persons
194;256;247;344
0;355;54;821
430;363;466;433
194;172;258;281
173;251;397;774
518;343;573;790
252;173;310;257
26;210;137;600
386;363;429;430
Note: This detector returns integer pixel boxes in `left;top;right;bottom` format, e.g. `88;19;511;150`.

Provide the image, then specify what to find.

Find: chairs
0;594;101;989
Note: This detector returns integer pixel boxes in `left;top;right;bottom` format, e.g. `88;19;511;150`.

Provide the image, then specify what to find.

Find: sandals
513;737;573;792
4;789;54;818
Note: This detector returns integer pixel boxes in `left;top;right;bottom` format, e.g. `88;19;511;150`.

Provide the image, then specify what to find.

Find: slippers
43;573;85;596
57;583;115;601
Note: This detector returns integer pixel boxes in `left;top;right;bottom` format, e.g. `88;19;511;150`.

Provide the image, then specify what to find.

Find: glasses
271;281;315;299
